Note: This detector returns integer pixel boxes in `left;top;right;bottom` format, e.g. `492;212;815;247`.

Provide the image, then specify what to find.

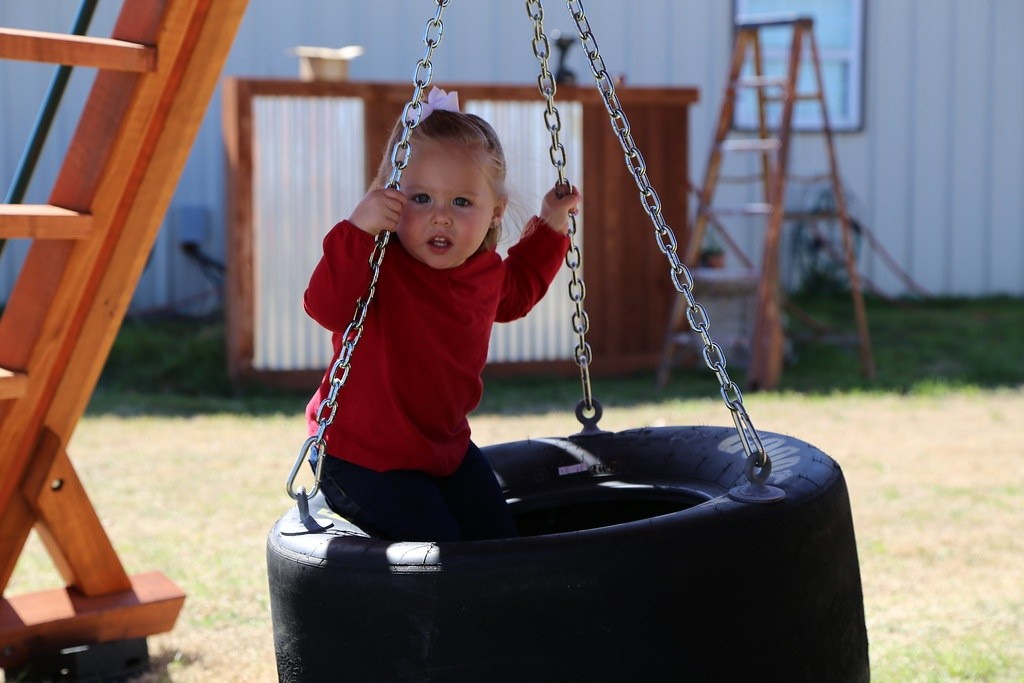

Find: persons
303;85;583;539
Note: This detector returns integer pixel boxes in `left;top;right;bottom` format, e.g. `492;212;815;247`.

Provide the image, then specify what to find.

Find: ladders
658;16;879;396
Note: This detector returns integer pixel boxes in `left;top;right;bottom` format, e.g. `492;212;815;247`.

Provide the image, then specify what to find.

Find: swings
263;3;875;683
